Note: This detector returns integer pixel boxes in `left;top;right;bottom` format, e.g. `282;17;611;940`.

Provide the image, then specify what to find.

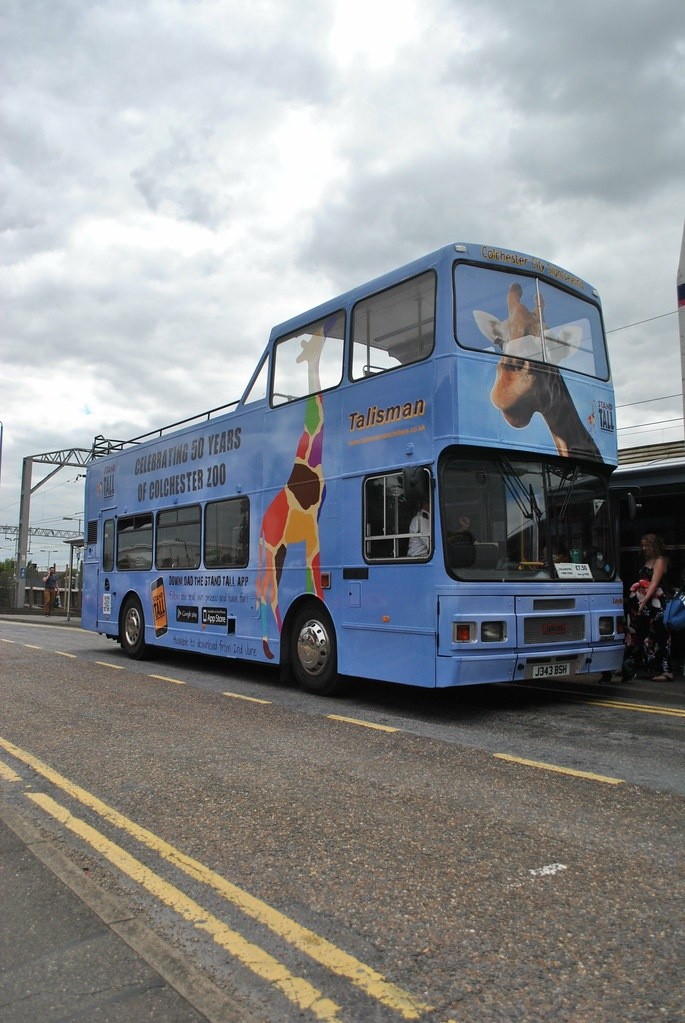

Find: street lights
62;517;80;536
40;550;58;568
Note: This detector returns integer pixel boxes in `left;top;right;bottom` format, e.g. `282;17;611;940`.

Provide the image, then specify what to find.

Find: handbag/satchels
663;583;685;635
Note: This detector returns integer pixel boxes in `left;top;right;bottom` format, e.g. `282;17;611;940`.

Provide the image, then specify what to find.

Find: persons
614;534;673;681
598;658;636;684
42;567;58;617
407;498;469;557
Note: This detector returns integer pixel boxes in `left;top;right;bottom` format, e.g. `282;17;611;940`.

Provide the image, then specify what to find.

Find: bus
78;243;625;698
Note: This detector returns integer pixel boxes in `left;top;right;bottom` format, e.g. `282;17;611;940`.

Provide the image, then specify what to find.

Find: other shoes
598;674;612;684
651;673;674;682
621;671;637;685
